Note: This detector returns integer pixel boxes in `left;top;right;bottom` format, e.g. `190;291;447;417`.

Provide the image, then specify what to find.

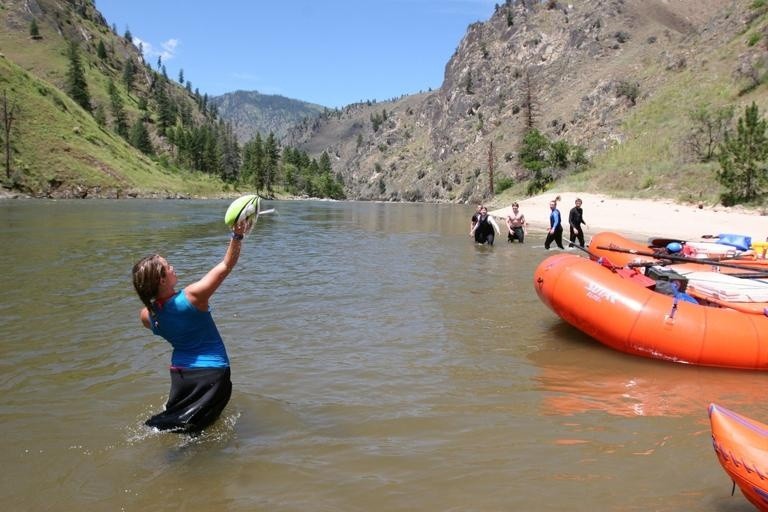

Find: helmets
666;242;683;253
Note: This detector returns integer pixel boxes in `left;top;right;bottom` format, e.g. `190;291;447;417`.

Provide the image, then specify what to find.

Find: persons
506;201;529;243
469;205;500;247
544;200;565;250
568;197;590;249
131;222;250;434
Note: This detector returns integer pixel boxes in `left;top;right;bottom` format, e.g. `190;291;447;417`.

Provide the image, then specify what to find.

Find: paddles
562;237;657;288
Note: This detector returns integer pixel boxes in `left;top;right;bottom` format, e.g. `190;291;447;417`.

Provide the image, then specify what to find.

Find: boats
533;230;767;372
704;401;768;512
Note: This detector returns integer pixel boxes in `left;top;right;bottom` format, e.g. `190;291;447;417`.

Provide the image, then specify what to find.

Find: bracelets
230;232;245;240
